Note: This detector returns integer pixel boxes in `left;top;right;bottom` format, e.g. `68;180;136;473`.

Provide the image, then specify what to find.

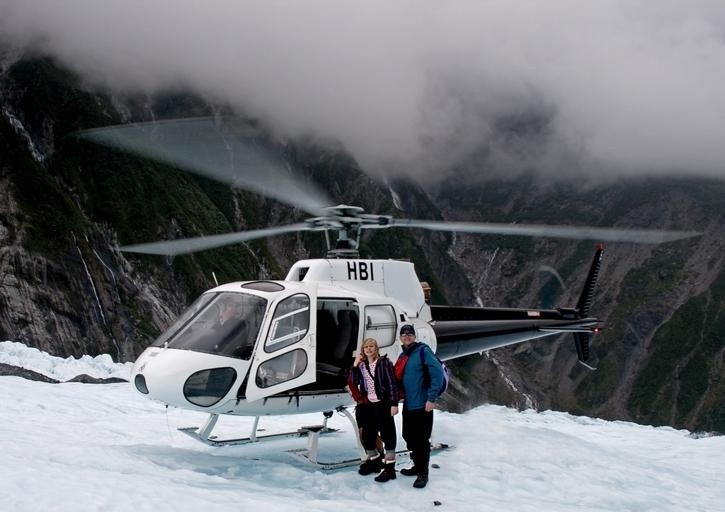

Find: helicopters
60;117;702;474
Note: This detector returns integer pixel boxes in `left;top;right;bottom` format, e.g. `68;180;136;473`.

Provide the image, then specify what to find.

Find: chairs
316;308;359;369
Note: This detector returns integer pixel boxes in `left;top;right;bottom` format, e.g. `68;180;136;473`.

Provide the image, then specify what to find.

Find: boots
359;451;396;483
400;462;429;488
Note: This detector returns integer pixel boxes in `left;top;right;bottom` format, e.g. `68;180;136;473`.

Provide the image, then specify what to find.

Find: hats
400;325;415;335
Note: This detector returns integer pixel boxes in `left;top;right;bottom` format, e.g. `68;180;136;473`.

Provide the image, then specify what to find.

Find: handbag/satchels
394;354;409;382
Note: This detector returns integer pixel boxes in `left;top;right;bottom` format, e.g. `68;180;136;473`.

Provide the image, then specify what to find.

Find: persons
393;325;444;488
350;338;399;482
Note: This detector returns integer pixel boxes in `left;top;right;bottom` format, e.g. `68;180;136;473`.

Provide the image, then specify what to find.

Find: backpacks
375;357;405;400
420;345;449;397
